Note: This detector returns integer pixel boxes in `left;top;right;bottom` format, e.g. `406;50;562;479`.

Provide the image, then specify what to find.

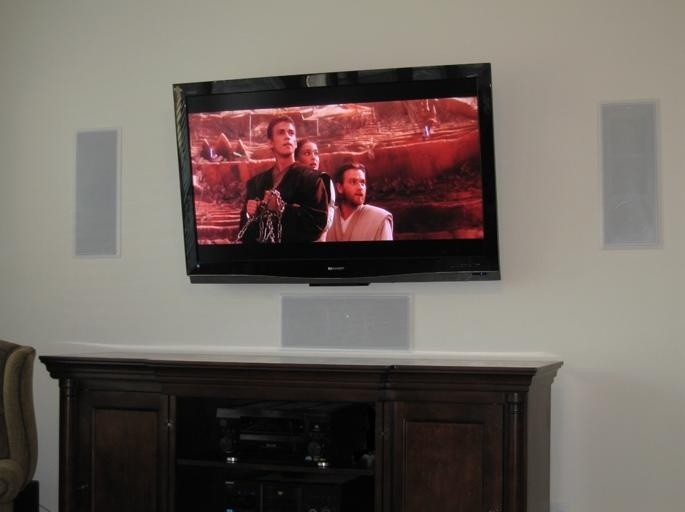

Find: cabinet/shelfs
36;349;569;512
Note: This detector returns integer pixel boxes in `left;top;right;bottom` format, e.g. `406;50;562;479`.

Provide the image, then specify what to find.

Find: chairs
0;337;44;511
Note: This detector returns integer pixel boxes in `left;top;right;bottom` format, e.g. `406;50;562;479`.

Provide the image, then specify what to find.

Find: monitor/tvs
174;61;500;285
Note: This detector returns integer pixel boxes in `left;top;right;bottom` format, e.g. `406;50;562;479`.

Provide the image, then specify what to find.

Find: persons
328;162;395;243
295;138;337;241
239;116;327;243
